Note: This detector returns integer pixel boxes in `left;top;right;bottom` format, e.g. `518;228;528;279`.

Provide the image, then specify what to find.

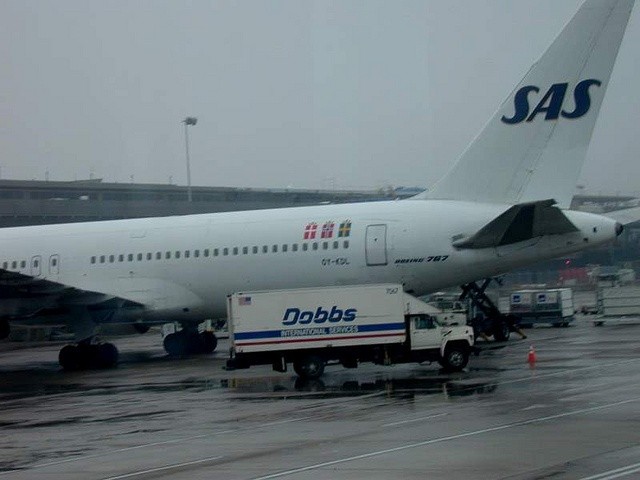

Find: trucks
497;288;574;327
225;283;475;380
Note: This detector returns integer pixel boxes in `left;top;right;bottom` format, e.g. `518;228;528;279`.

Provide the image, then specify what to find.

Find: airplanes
1;0;639;370
